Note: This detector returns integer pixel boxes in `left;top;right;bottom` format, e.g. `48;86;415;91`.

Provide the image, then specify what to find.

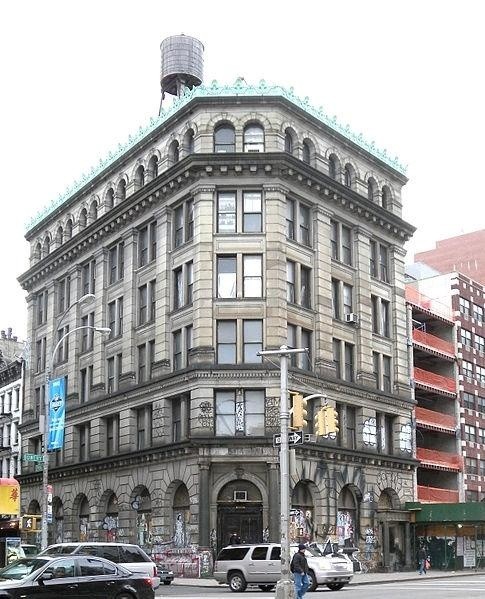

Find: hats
298;543;307;550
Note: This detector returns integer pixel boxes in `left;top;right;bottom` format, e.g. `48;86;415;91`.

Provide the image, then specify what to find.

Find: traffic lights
294;396;308;431
19;514;37;532
314;413;326;438
326;410;339;433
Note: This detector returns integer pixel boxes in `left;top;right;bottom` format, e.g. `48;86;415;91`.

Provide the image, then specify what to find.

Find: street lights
40;293;111;550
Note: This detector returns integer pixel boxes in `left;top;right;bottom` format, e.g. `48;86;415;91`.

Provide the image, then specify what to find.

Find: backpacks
289;553;297;574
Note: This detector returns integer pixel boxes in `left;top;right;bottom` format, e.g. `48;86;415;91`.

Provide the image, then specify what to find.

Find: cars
0;541;174;599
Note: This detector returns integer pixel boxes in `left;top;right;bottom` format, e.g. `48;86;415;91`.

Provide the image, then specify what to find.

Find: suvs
213;543;354;592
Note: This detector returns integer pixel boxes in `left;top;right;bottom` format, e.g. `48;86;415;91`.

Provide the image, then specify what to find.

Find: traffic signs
274;432;304;445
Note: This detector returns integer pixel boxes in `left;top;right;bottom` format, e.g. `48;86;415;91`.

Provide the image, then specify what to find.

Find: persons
416;546;427;575
228;532;242;545
293;544;310;599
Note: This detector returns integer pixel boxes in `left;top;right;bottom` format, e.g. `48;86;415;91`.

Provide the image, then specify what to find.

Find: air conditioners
345;313;358;323
